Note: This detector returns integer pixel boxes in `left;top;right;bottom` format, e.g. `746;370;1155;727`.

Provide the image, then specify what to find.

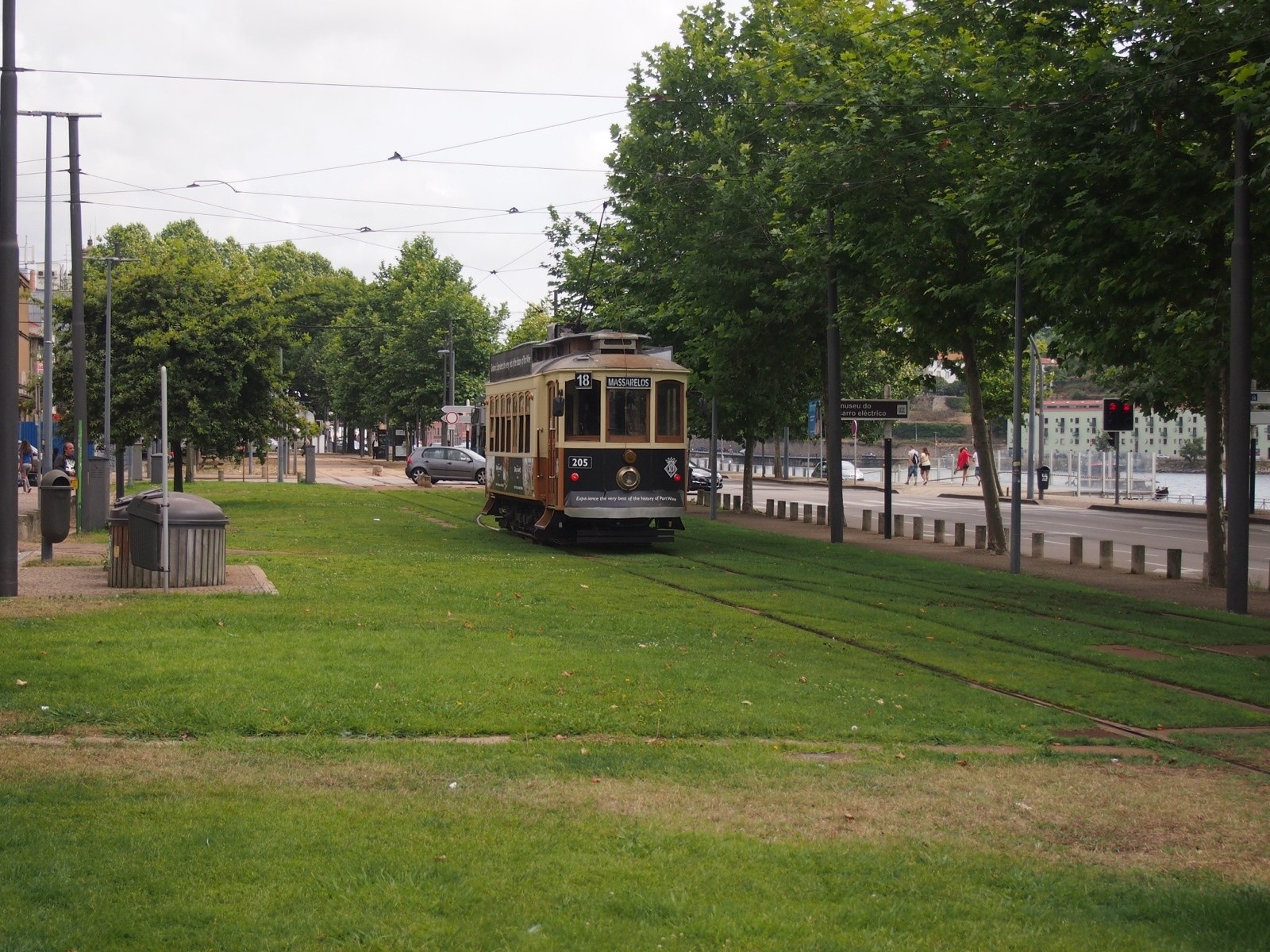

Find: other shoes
20;492;27;494
905;482;909;484
914;482;917;485
27;489;31;493
923;480;927;485
954;468;957;475
962;484;964;486
978;480;980;486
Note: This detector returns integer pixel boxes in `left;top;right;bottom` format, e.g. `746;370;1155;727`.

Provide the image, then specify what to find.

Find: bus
486;319;692;546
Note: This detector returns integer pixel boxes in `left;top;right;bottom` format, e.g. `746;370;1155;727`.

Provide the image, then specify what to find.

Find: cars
811;459;864;481
688;458;724;492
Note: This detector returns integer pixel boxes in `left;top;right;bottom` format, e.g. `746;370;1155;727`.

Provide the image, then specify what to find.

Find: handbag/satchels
919;464;921;468
964;463;969;469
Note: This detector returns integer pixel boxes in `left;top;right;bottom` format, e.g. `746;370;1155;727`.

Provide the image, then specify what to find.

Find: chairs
1156;488;1168;499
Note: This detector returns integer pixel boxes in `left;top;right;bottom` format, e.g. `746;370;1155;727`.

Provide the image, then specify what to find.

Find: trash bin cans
1037;466;1050;489
127;495;161;571
42;469;73;543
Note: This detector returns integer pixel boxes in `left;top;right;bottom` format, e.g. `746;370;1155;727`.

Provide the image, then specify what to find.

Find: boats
1069;454;1170;503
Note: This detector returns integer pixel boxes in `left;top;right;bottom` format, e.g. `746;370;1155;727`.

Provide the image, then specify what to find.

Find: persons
372;436;380;459
904;444;919;485
1137;485;1164;491
973;451;981;486
54;442;76;476
920;447;931;486
19;440;32;494
954;446;972;486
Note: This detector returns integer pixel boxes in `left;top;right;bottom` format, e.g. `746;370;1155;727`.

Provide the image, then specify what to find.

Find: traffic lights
1102;396;1135;432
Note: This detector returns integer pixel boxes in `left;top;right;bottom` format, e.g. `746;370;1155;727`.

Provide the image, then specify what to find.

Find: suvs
406;447;487;486
15;440;58;486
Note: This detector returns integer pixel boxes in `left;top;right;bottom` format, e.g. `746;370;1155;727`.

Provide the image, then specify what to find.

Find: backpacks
912;453;919;464
